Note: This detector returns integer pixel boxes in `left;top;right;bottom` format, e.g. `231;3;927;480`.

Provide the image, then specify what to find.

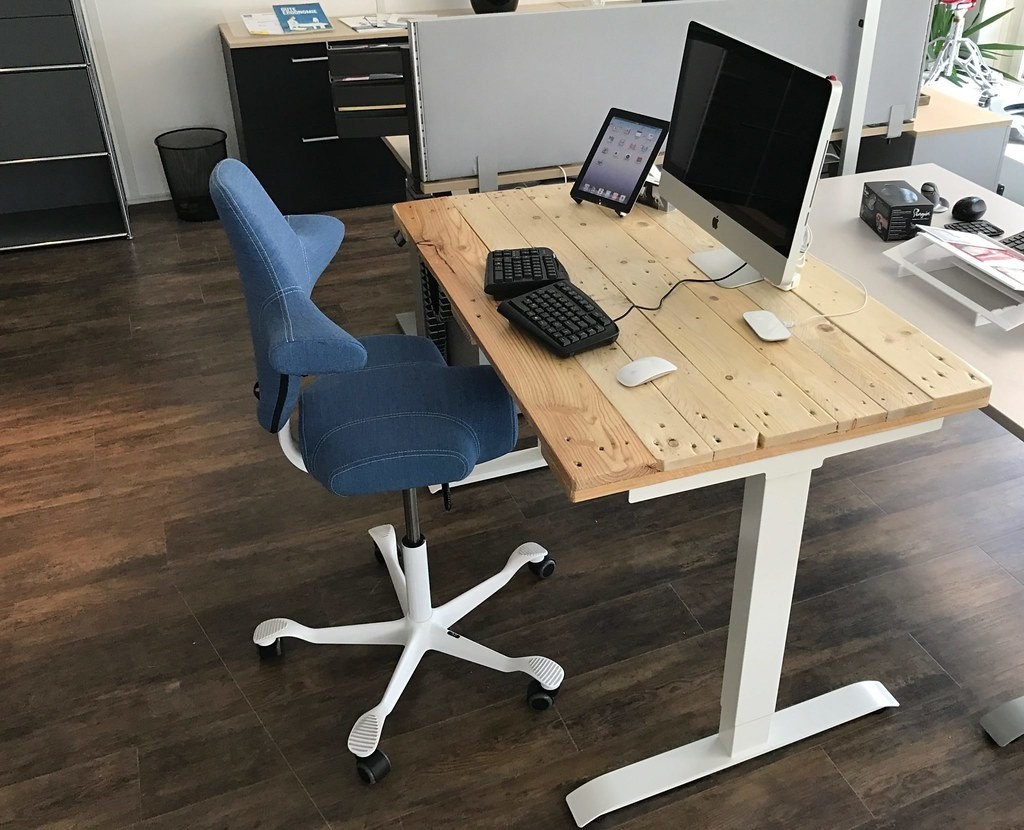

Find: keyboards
943;220;1024;256
485;246;620;357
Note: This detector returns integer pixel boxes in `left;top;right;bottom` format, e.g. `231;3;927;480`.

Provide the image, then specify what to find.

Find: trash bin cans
153;128;229;222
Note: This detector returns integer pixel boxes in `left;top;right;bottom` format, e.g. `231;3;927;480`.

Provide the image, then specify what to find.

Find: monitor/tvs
660;20;843;287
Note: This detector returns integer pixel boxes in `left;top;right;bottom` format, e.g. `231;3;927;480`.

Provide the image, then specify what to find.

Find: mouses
952;196;987;222
615;356;677;387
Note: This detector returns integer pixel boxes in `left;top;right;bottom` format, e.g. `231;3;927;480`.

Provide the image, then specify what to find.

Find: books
241;12;284;35
273;2;334;34
338;13;437;34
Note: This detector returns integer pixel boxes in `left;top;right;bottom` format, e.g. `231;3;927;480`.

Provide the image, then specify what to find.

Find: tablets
570;107;669;213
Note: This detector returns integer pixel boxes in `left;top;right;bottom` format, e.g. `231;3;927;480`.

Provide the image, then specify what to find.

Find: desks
219;0;1024;828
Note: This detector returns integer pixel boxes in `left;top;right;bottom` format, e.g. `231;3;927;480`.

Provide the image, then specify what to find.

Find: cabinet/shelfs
0;0;134;252
325;41;409;141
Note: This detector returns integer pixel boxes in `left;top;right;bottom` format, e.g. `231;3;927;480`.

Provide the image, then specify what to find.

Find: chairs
210;158;564;786
